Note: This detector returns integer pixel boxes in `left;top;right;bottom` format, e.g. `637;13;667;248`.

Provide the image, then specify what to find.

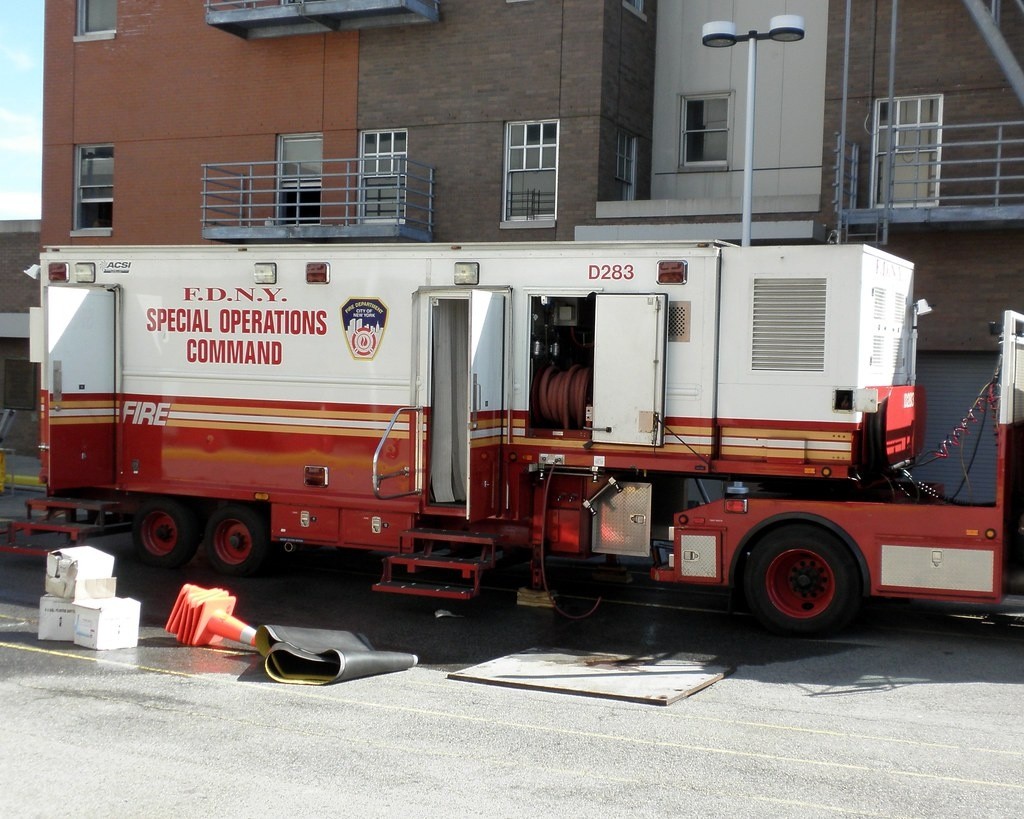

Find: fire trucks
0;234;1023;643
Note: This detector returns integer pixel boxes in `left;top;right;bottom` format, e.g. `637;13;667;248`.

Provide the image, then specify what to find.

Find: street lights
700;11;807;249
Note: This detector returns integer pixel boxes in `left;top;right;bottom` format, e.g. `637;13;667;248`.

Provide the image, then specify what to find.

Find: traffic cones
163;581;258;649
